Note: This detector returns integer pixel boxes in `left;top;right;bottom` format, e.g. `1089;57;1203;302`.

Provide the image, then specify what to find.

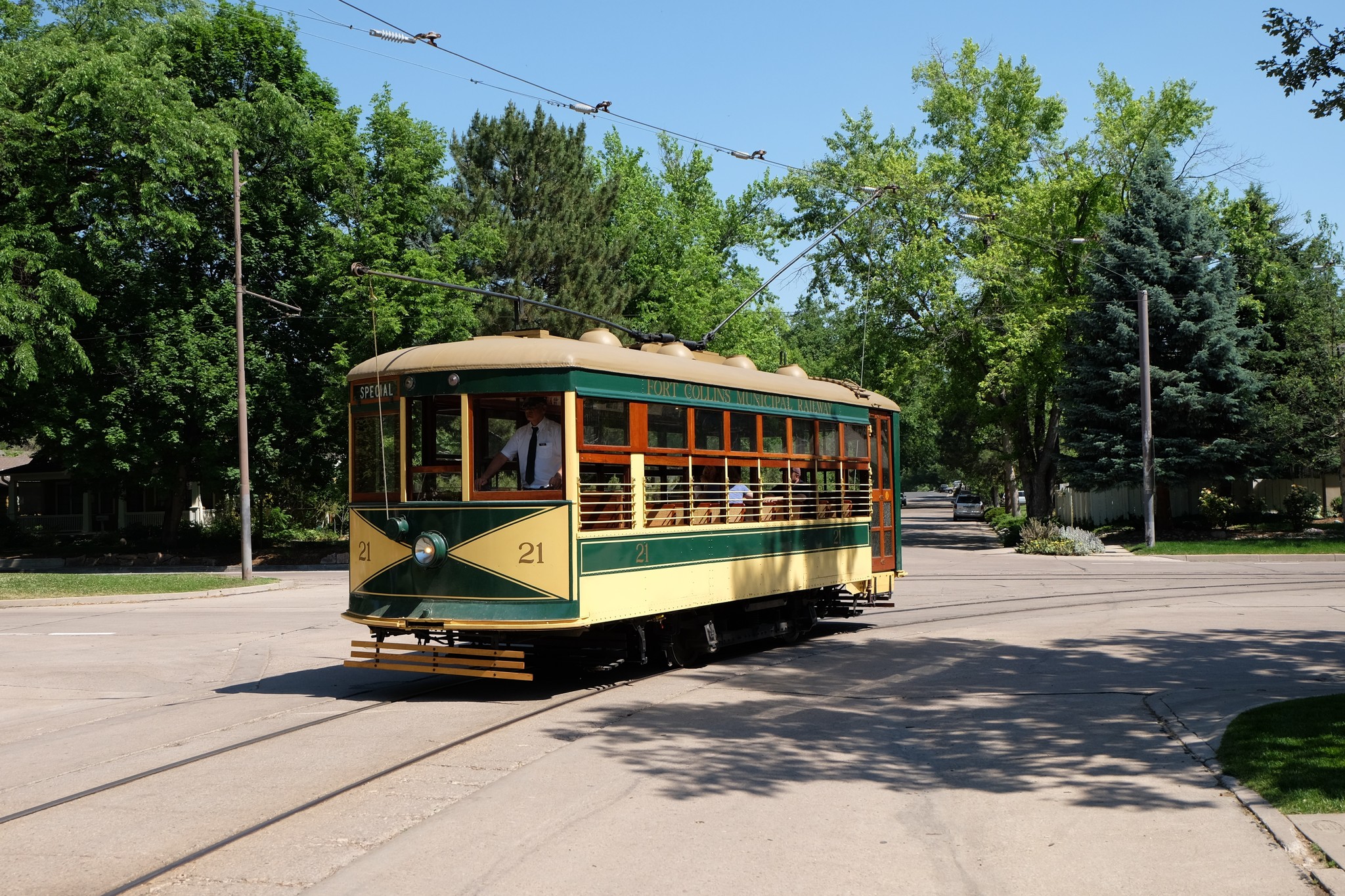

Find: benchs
646;496;854;526
581;490;624;529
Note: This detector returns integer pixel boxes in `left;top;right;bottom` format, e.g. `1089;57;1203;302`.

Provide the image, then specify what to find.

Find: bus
339;185;905;693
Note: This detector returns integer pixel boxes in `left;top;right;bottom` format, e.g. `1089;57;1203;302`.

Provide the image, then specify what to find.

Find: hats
522;396;547;406
779;468;801;477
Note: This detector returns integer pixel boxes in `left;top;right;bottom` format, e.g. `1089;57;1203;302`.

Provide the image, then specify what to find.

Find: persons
727;467;753;504
694;465;720;502
762;467;811;518
476;398;562;490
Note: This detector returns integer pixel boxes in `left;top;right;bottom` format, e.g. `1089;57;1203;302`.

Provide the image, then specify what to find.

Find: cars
900;493;907;506
951;493;984;522
944;480;971;502
939;484;949;493
1002;490;1026;505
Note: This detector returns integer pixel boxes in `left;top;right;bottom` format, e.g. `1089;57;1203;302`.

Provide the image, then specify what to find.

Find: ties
525;427;539;485
